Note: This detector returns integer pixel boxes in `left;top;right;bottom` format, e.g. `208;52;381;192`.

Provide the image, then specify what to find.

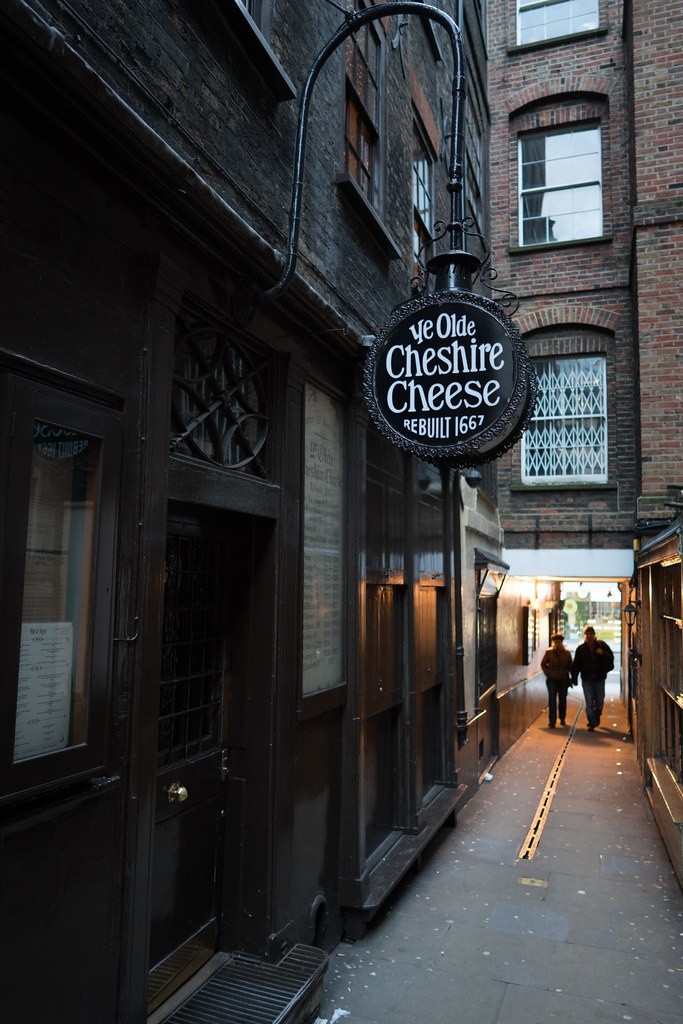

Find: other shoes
587;723;594;732
560;720;565;725
548;723;555;727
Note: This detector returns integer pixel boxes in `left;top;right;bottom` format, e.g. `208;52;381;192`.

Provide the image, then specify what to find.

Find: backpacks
595;638;614;672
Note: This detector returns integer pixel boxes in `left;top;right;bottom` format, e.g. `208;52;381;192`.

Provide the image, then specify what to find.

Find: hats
551;634;564;640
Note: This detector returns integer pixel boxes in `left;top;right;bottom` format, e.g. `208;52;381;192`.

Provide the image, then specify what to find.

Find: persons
571;626;615;731
539;632;576;728
